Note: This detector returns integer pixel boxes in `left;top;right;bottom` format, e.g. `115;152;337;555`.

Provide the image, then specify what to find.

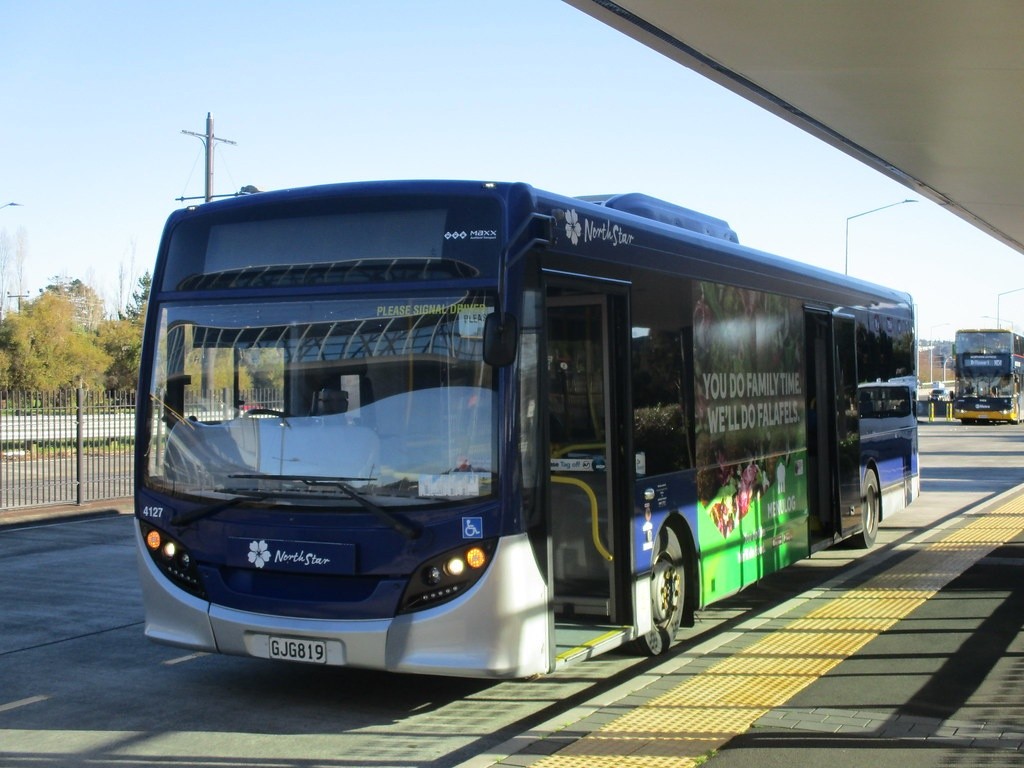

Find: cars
927;388;949;402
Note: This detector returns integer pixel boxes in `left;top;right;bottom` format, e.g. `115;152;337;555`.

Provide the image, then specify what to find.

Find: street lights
943;356;952;381
982;315;1013;333
844;199;920;275
929;322;951;383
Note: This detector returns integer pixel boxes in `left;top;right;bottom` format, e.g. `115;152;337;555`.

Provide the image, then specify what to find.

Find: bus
856;376;917;438
128;175;922;681
949;329;1024;427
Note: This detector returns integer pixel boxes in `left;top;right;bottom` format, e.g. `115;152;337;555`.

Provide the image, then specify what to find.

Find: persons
965;380;975;395
